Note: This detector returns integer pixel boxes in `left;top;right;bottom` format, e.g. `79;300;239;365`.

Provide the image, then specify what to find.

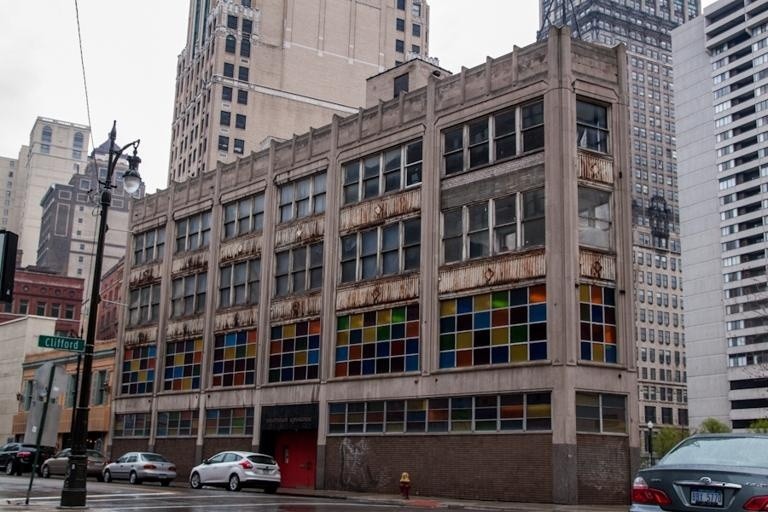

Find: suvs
188;449;283;493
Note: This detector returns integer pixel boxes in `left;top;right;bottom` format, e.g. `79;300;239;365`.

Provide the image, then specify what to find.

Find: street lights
645;419;656;468
54;119;144;511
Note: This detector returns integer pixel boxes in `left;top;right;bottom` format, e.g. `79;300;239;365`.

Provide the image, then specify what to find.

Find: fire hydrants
399;471;411;500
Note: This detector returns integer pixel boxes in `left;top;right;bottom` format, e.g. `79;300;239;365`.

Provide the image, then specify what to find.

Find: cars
0;442;56;477
41;444;112;484
625;427;768;511
102;450;177;489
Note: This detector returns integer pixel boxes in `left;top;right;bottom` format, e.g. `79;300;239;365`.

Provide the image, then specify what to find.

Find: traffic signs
37;334;88;352
